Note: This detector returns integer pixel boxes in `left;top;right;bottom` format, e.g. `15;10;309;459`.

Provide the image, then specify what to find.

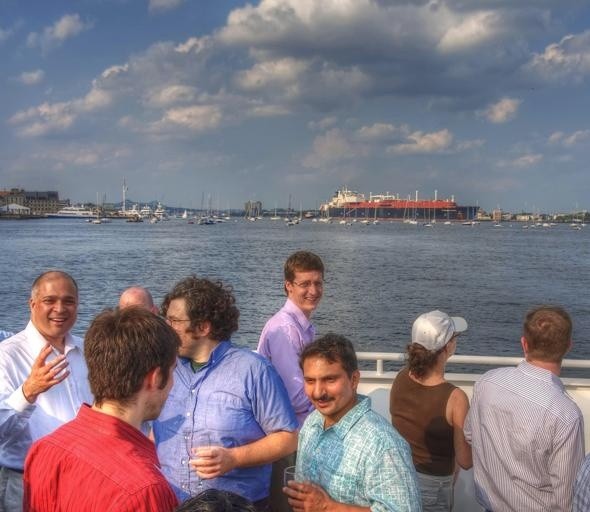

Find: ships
45;175;144;219
320;185;482;219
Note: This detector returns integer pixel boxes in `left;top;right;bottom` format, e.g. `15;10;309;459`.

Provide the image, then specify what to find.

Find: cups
283;464;314;495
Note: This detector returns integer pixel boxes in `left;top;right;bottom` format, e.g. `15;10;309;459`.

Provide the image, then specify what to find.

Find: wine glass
182;431;216;492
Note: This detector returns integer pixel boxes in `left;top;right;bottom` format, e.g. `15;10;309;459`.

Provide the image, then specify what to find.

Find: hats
411;310;468;353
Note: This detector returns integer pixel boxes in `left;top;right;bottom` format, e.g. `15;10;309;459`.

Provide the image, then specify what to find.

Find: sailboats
91;192;101;224
307;194;386;228
400;198;480;226
493;200;589;231
179;191;305;228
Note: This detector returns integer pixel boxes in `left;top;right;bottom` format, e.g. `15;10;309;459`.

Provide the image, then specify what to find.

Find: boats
100;217;112;222
128;199;170;224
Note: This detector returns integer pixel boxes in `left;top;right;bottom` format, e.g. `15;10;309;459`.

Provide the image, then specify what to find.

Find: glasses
164;318;190;327
291;279;326;287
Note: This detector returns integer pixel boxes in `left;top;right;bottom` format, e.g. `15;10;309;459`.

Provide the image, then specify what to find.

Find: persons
174;488;257;512
0;271;95;512
141;276;298;512
256;250;323;512
390;310;473;512
462;305;585;512
281;333;422;512
21;306;182;512
119;286;159;316
